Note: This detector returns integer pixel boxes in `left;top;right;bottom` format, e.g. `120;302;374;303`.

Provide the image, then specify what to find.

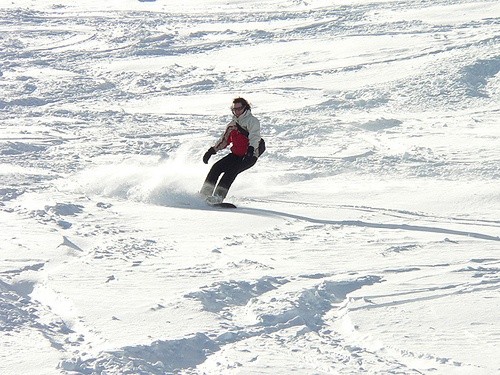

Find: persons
198;97;266;204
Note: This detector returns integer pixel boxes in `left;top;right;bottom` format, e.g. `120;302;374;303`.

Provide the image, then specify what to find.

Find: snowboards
207;203;237;208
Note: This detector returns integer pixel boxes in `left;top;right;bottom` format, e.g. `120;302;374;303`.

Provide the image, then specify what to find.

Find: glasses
232;103;247;112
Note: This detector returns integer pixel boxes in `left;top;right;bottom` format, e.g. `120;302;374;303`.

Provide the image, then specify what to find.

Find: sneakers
191;192;223;205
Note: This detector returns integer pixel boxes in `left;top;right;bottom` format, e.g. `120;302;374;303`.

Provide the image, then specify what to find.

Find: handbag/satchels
258;138;266;157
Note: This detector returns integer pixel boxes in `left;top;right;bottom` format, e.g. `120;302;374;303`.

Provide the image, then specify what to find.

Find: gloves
203;147;217;164
242;146;254;163
